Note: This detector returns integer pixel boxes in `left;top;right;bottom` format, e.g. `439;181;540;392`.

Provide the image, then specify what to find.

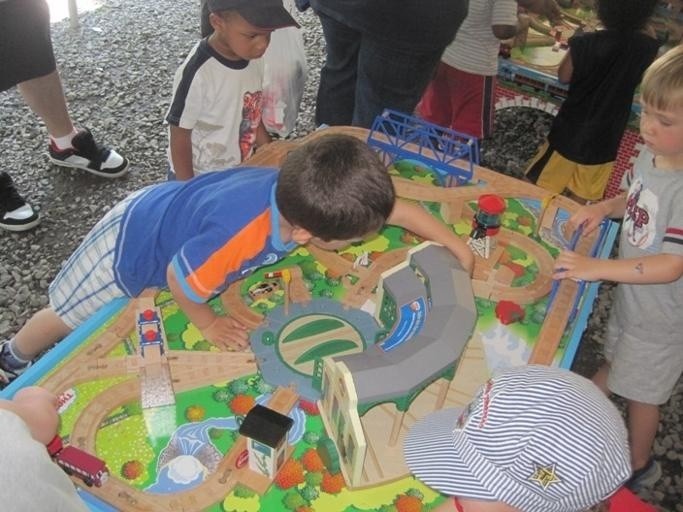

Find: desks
495;0;683;206
0;124;618;512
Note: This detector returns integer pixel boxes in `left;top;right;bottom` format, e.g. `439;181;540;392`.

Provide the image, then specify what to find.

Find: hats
206;0;302;30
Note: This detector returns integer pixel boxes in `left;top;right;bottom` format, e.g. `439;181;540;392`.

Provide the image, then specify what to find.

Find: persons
538;1;671;203
549;44;682;503
0;127;475;387
0;386;88;512
422;1;533;142
0;0;132;230
291;0;468;134
160;2;307;181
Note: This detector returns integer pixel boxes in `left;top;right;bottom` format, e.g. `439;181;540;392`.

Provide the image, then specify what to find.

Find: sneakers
0;168;42;232
0;338;32;387
624;456;663;495
46;127;130;179
